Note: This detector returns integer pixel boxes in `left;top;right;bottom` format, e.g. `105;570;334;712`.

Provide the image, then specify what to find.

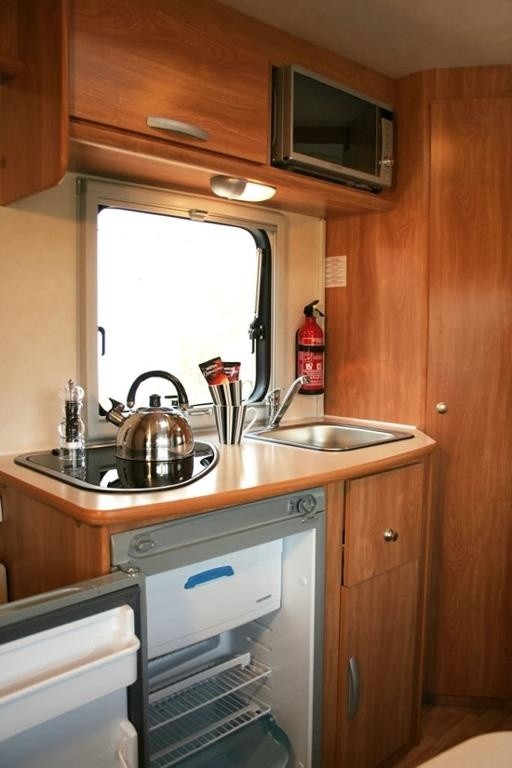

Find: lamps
209;175;277;204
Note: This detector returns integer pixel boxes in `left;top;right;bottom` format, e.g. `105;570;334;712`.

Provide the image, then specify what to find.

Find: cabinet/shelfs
429;68;512;699
2;0;274;204
330;459;428;767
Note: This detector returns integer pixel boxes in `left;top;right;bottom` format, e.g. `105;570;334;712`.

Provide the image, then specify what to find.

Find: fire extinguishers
296;298;327;395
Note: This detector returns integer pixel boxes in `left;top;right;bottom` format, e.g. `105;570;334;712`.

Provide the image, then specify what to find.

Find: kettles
106;369;196;463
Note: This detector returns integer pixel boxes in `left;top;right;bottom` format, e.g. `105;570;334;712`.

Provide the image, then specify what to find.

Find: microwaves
270;61;396;195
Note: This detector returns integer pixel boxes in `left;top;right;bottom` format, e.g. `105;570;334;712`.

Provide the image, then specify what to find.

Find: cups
207;379;258;446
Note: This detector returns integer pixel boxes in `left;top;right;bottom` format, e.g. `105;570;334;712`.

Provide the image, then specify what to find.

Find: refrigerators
0;483;330;768
